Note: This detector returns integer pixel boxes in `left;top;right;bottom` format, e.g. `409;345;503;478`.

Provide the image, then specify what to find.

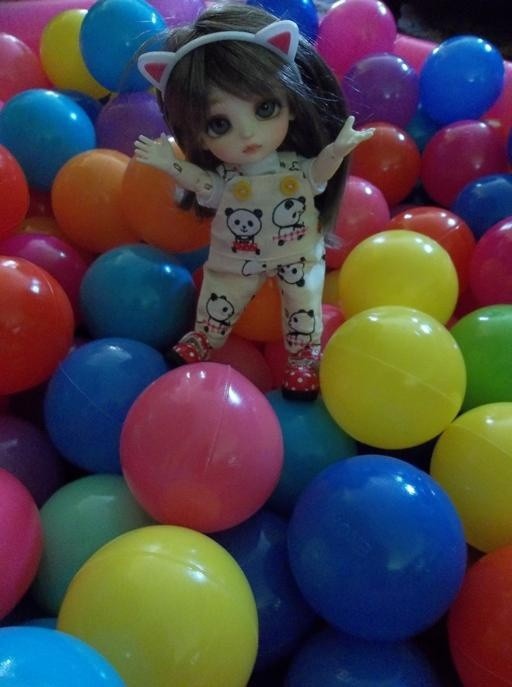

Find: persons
135;7;376;403
